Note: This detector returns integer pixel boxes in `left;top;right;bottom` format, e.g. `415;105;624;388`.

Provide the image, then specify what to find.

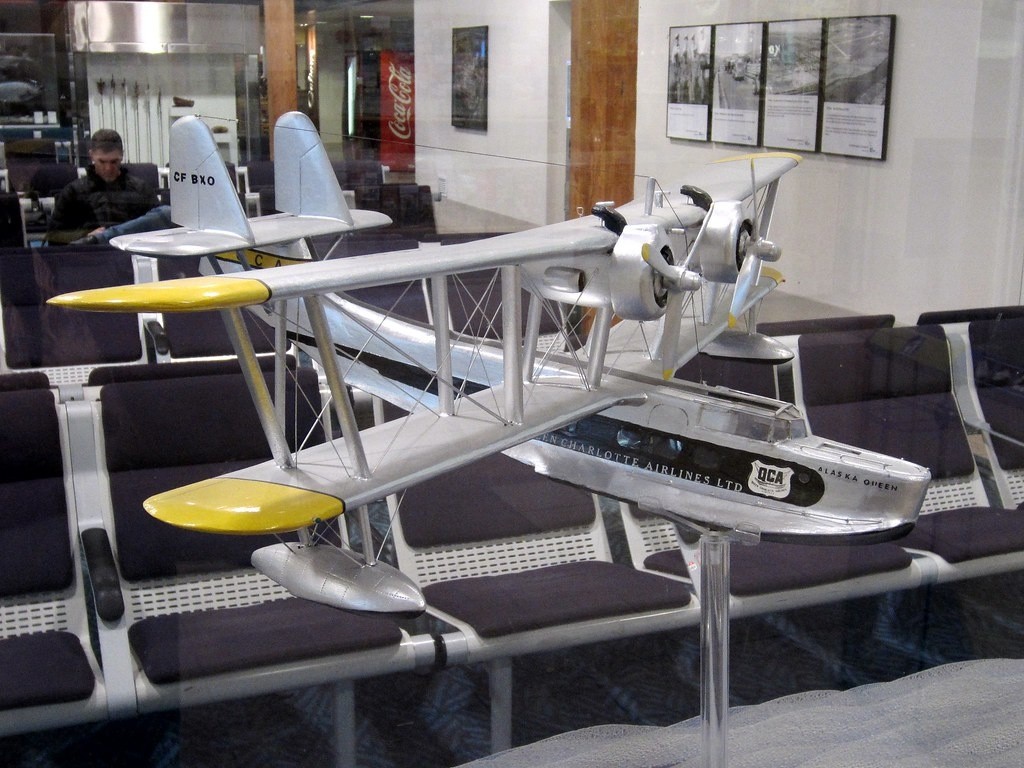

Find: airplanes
46;108;933;622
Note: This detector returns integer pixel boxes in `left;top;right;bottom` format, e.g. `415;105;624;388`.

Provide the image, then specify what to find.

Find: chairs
0;137;1024;768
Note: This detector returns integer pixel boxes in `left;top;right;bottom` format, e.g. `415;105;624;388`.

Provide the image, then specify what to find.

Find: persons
46;128;178;246
